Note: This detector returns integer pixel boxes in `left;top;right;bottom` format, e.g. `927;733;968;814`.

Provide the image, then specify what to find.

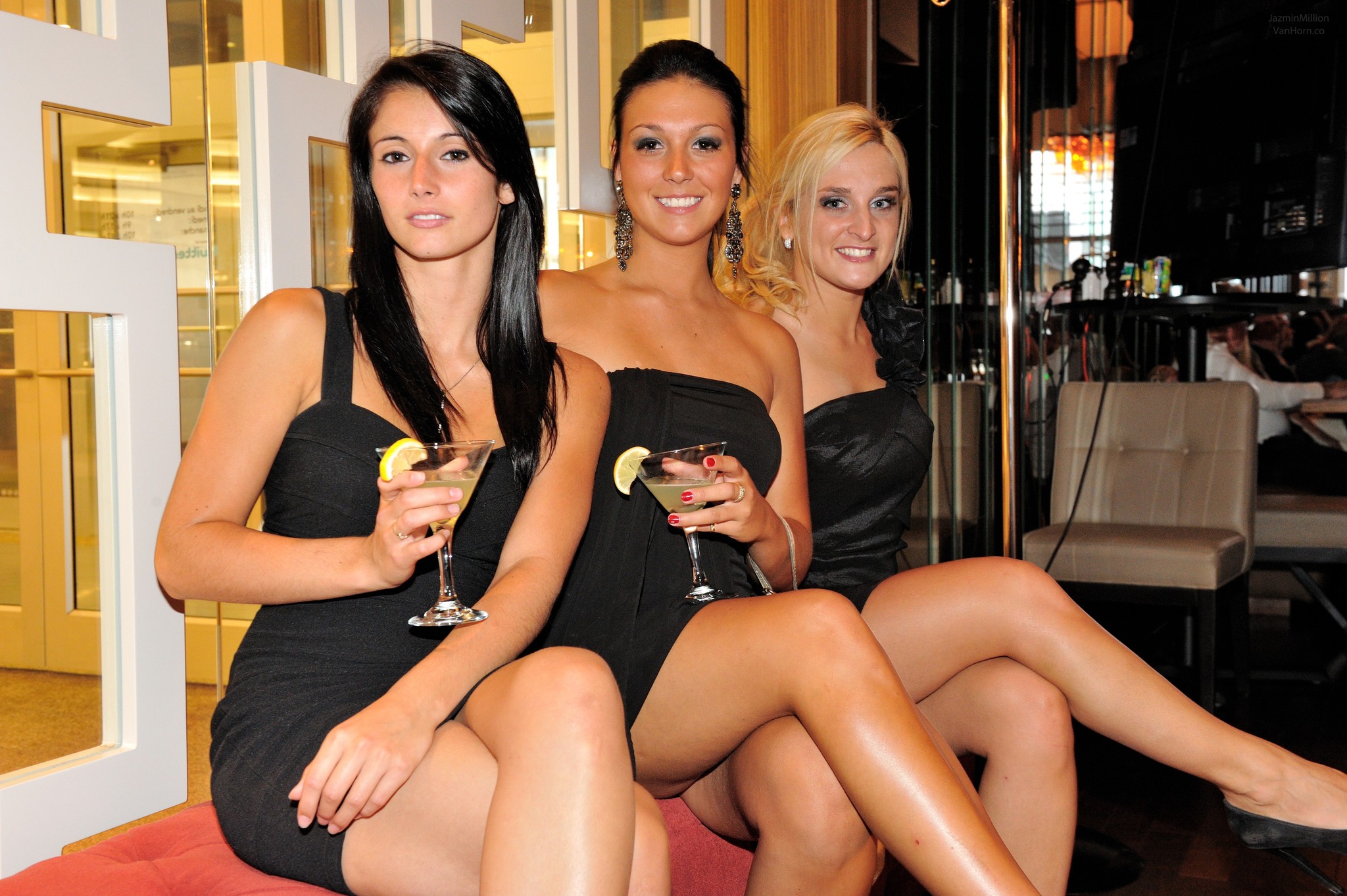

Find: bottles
900;258;980;307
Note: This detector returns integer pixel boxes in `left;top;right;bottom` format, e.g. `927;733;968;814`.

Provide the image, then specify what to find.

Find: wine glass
375;439;489;627
629;441;741;604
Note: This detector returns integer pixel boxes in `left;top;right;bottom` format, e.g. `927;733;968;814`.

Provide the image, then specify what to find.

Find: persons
153;39;670;896
727;104;1347;896
1024;311;1109;479
1176;282;1347;496
514;40;1044;896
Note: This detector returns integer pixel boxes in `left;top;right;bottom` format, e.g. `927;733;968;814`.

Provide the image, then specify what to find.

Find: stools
1255;494;1347;689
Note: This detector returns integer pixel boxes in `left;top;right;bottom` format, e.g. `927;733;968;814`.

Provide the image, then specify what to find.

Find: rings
392;521;408;539
710;524;715;533
729;482;745;503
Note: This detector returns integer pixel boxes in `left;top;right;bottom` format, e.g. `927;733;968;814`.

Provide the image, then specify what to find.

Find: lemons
379;438;428;481
613;446;651;495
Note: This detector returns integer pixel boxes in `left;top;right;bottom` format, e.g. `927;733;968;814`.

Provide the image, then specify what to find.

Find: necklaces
430;349;487;433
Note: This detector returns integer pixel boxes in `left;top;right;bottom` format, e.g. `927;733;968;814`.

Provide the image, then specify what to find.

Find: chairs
1021;378;1260;715
895;381;991;572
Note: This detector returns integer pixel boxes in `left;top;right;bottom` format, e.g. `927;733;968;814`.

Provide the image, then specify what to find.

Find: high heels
1222;799;1347;896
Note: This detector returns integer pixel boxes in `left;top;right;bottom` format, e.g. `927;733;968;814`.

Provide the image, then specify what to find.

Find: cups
980;292;999;307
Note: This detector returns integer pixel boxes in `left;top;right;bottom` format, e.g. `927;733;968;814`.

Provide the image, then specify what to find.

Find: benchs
0;798;753;896
